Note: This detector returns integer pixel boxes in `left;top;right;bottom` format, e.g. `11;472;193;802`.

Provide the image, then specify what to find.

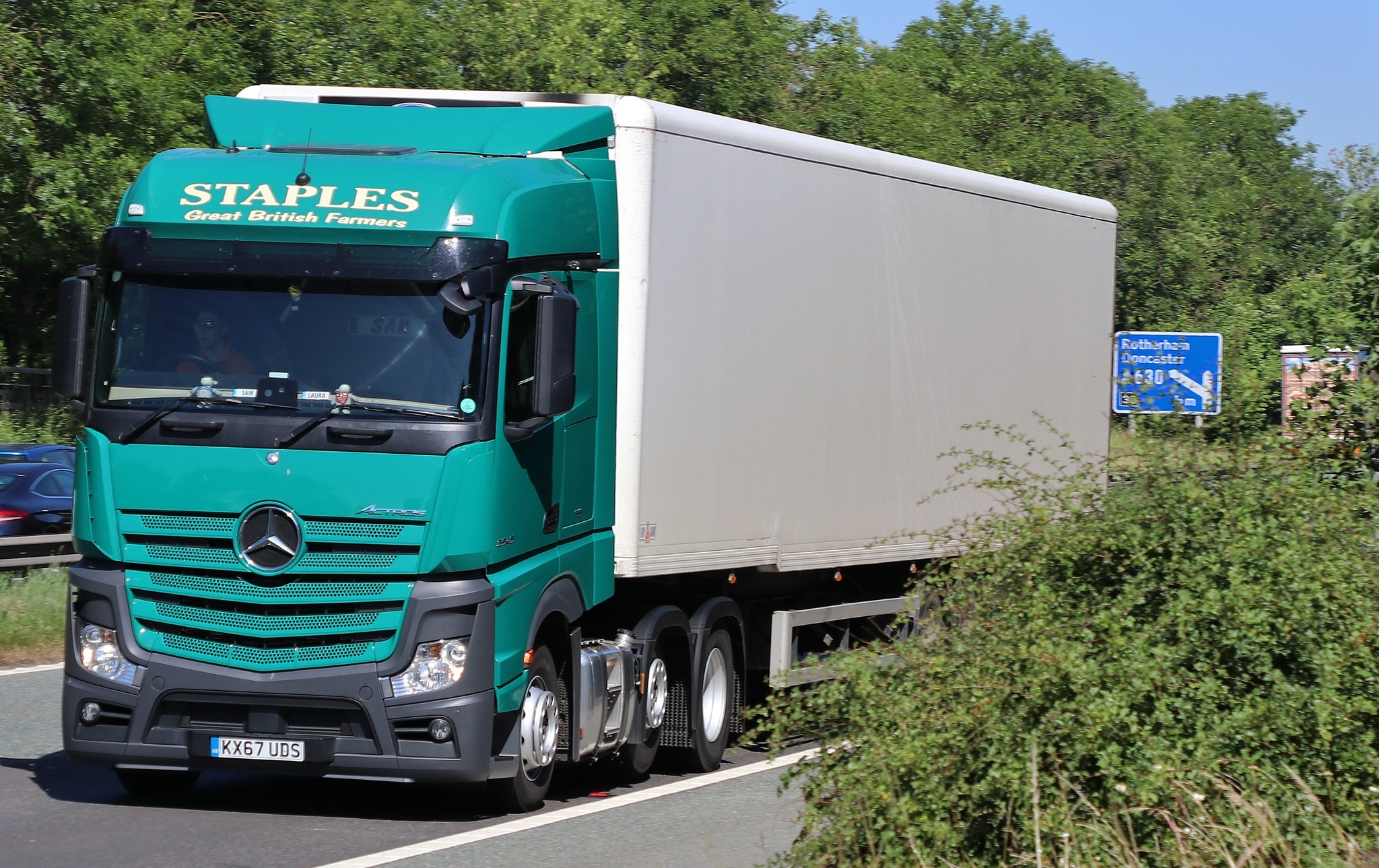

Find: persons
176;311;253;375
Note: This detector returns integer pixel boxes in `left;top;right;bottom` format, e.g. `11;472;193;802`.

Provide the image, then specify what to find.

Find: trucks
1280;344;1362;451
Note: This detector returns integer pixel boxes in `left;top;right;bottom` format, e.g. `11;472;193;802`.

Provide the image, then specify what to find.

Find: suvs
0;443;77;471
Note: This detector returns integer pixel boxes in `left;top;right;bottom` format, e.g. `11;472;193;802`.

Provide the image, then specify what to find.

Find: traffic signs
1112;330;1223;416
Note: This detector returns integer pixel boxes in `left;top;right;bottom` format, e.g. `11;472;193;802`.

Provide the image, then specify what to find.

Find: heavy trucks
55;83;1118;815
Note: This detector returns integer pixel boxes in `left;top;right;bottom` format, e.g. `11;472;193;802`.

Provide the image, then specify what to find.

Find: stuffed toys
330;384;357;414
188;376;221;408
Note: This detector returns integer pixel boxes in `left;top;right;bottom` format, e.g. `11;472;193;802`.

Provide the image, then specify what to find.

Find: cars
0;462;75;564
